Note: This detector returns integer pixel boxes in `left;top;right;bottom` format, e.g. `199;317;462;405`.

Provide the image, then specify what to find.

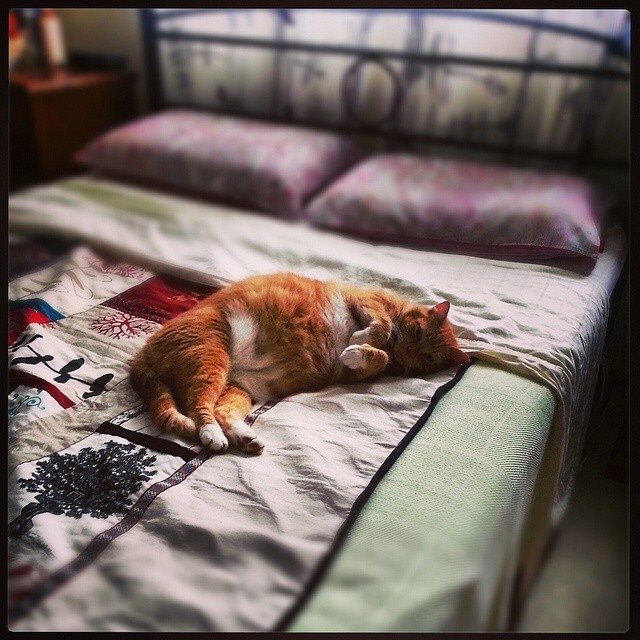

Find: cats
130;273;471;456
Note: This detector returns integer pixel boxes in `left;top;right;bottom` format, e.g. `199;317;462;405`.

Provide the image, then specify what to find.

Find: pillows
304;149;628;269
70;103;355;218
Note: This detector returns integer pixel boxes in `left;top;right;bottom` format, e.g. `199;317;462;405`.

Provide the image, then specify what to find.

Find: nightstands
8;66;138;178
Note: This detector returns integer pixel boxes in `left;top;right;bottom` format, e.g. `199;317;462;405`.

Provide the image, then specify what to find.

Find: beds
5;3;634;636
